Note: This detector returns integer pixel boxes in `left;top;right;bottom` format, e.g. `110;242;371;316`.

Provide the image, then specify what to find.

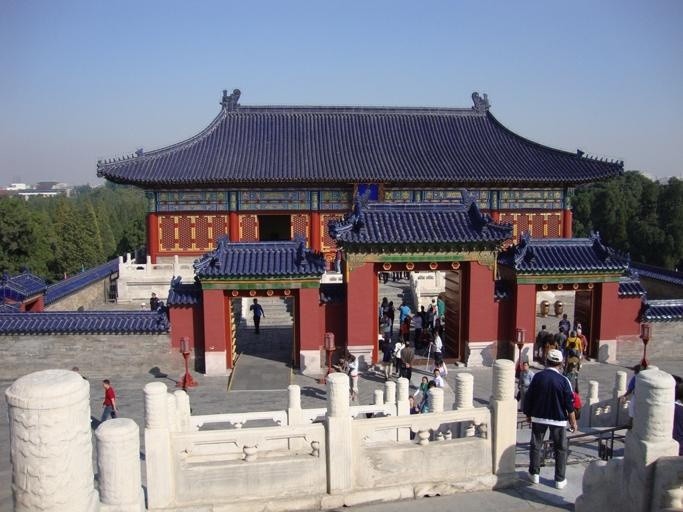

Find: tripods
419;342;433;371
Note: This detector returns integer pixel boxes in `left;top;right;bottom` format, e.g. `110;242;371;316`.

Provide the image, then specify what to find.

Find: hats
546;348;563;362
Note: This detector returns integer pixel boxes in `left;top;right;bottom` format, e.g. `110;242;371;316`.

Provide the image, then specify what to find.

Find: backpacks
569;342;576;348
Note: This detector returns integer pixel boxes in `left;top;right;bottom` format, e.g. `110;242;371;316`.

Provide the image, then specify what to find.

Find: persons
378;270;413;284
331;352;358;401
139;292;164;311
518;314;587;488
617;364;645;433
374;294;448;441
249;298;265;333
97;378;121;426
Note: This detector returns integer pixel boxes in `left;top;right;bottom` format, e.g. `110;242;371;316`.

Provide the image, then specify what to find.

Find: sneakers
555;477;568;489
527;471;540;484
